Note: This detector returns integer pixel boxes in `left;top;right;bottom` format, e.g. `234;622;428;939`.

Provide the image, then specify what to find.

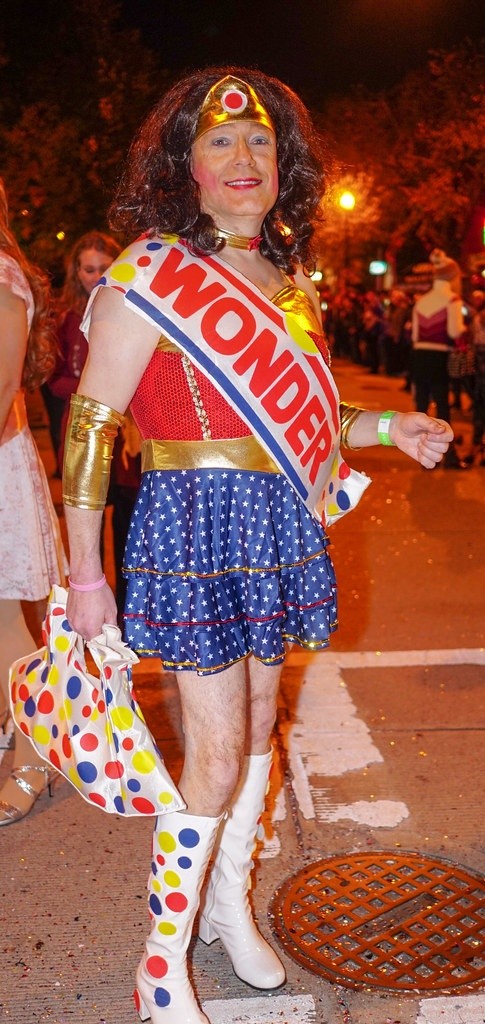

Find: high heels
0;763;59;826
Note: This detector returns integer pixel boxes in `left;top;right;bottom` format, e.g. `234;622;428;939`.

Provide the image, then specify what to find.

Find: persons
0;178;485;826
63;69;454;1024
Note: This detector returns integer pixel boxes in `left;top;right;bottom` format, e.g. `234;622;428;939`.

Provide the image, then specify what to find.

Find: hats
429;250;459;283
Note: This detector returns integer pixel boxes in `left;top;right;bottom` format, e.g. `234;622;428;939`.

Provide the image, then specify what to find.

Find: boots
199;743;287;991
127;809;228;1024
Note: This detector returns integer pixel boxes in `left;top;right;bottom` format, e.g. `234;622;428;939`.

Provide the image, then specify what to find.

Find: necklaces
214;230;261;251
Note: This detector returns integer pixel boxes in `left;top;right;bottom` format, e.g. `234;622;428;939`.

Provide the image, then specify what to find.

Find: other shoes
462;456;474;464
445;457;468;471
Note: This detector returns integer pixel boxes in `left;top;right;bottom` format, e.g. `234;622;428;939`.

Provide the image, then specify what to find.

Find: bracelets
378;411;397;446
68;573;105;591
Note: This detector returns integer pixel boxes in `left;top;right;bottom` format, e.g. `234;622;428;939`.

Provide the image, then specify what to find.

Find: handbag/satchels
448;342;475;377
7;586;187;818
